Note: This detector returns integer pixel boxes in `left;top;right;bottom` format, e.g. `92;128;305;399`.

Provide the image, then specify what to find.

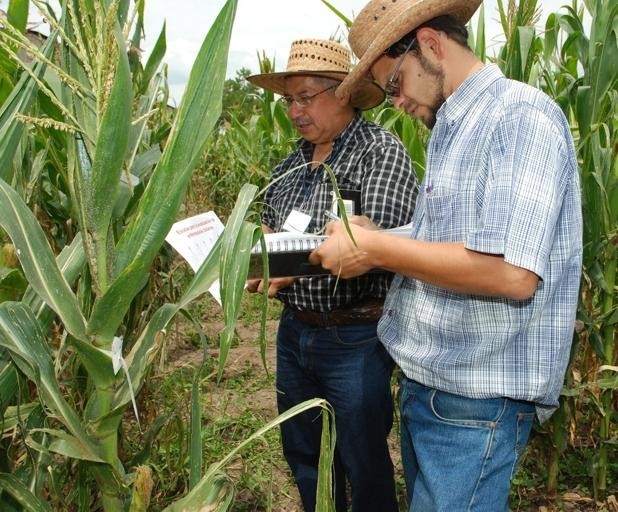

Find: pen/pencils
320;208;343;222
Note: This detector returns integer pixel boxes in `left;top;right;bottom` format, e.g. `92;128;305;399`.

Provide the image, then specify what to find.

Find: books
244;222;416;276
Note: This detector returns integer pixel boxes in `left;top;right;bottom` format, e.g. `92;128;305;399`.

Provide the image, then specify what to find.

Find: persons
244;38;416;509
309;0;585;509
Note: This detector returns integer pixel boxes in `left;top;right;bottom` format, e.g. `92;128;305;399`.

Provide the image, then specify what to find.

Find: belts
290;299;384;328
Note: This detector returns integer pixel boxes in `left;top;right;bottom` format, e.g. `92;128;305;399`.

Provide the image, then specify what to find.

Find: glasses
383;38;416;99
280;83;340;106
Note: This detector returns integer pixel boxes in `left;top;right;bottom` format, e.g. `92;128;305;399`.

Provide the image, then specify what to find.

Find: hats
245;36;386;112
333;0;484;101
